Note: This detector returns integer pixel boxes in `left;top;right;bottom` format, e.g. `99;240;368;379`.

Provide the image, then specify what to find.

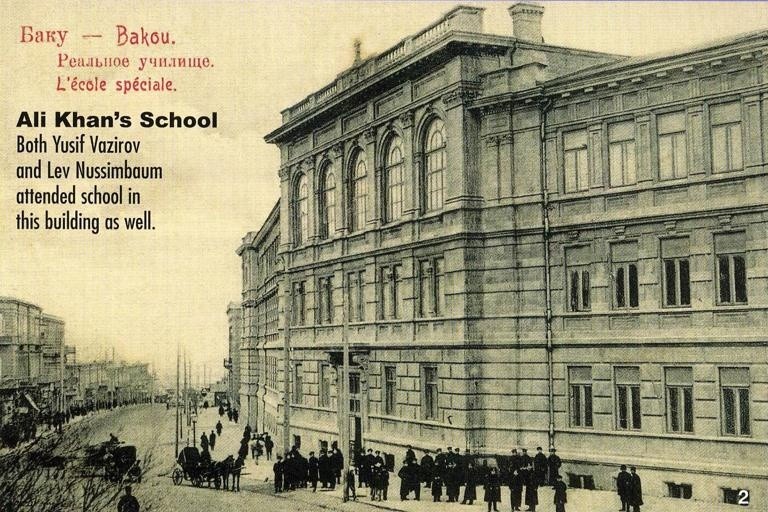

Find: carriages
171;445;247;493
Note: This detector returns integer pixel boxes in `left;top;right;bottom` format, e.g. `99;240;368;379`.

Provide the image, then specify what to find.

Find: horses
207;454;234;490
223;454;245;492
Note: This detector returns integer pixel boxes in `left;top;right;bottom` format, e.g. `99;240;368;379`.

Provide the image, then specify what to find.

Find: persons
616;464;631;512
625;466;643;512
1;391;569;512
